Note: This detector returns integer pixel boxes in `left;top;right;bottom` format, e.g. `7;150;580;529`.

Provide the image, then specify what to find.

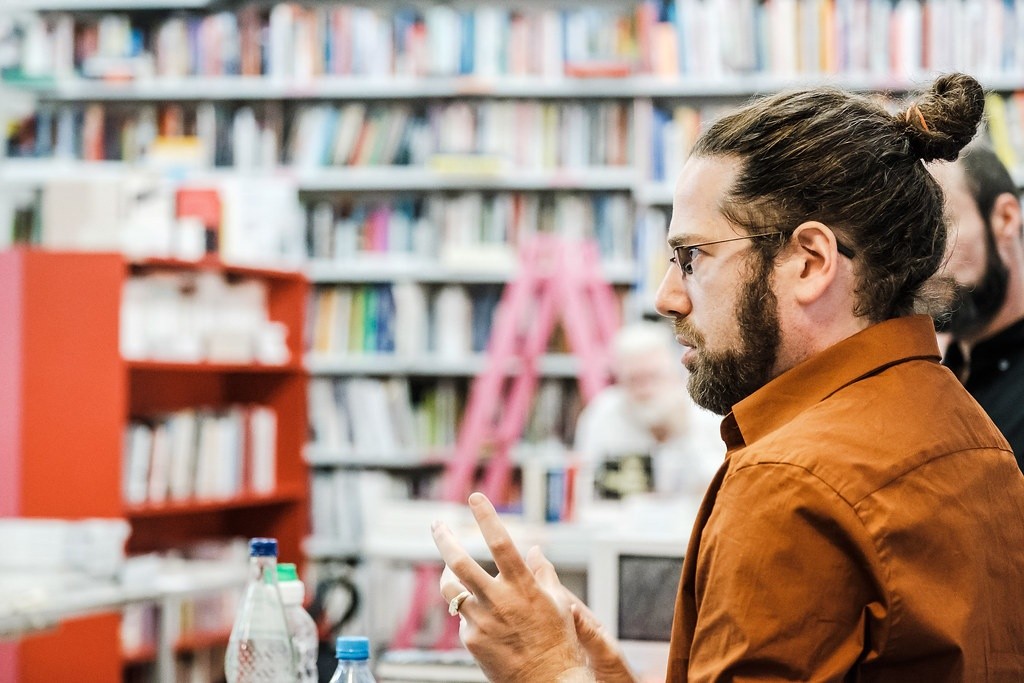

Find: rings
446;591;470;617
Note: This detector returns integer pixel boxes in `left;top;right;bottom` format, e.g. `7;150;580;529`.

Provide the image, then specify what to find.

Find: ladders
388;228;626;649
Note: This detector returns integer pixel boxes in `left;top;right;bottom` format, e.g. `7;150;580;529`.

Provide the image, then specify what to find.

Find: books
307;468;578;543
306;378;583;443
111;273;294;367
285;90;1024;170
317;563;590;681
304;0;1022;82
118;538;256;683
123;401;280;504
300;188;636;264
302;281;630;357
1;517;131;634
2;10;307;274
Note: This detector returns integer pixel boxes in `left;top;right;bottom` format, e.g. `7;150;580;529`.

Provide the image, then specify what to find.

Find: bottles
226;539;376;683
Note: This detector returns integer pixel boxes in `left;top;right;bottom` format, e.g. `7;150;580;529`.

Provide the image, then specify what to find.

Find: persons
913;140;1024;472
429;71;1024;682
567;321;731;526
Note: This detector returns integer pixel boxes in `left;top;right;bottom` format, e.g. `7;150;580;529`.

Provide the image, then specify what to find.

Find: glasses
669;230;856;274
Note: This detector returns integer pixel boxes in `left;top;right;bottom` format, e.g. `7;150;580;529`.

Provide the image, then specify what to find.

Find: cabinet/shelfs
0;0;1024;683
